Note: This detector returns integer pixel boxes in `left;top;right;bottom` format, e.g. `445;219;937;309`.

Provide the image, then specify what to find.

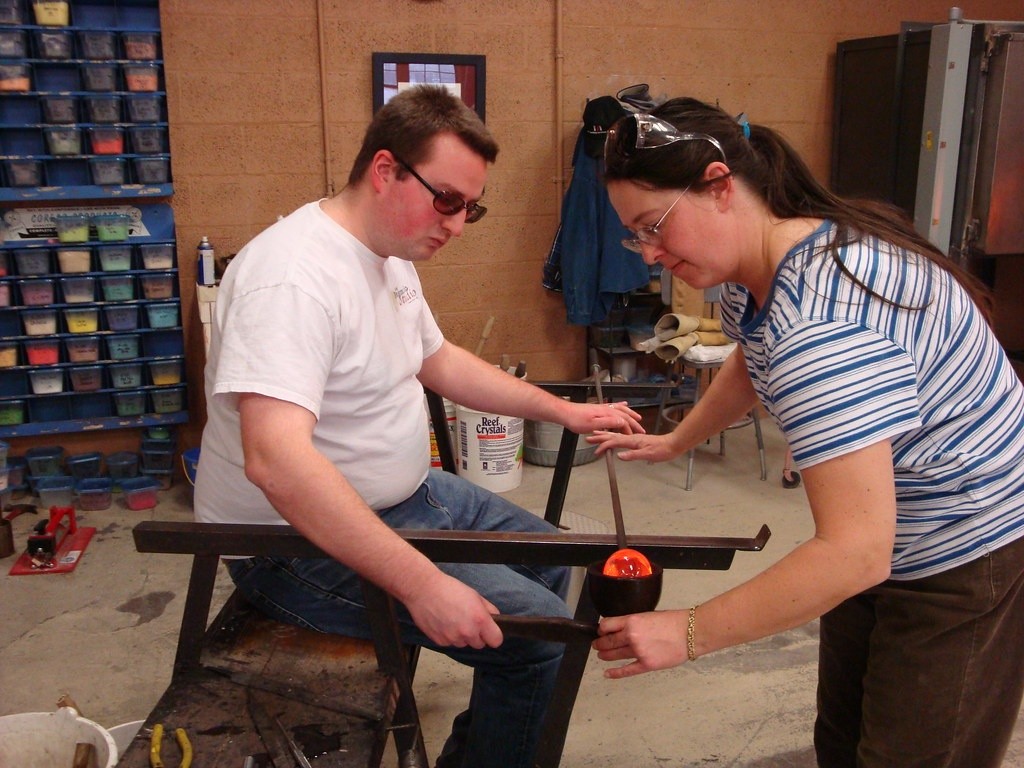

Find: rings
609;404;614;408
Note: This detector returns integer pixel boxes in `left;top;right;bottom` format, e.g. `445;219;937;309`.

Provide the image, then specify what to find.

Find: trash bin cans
0;707;119;768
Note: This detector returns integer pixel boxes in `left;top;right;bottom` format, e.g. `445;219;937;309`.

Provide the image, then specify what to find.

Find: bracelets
687;604;699;661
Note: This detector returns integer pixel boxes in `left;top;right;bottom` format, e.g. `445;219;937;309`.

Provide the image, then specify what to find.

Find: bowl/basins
524;444;604;466
586;559;664;618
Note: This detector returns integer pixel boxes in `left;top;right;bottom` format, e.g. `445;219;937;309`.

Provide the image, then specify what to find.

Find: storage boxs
591;271;697;407
0;0;187;511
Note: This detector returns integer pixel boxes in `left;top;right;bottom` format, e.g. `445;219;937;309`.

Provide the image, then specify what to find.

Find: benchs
105;580;429;768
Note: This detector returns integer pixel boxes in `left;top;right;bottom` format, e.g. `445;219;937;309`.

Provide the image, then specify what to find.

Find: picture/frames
371;52;486;127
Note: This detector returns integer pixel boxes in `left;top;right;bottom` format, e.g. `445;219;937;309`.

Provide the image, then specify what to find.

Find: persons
584;96;1024;768
194;84;646;768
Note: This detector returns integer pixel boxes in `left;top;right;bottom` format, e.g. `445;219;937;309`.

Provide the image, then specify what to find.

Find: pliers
150;723;193;768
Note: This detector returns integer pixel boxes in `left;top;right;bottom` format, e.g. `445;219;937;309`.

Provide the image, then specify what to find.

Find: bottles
197;236;216;286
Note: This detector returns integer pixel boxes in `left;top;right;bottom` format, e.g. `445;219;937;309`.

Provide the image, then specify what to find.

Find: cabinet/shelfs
0;237;190;438
582;258;683;417
0;0;175;200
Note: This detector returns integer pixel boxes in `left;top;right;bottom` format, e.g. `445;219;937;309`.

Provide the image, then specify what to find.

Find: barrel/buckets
452;364;527;493
0;706;147;768
423;393;457;472
533;418;598;449
181;447;200;486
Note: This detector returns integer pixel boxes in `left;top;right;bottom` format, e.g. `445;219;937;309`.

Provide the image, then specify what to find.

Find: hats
582;95;626;160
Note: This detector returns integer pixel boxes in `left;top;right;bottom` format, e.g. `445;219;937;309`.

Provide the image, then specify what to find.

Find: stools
652;358;766;490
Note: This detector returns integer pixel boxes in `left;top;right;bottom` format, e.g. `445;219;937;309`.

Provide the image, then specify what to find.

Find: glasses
622;176;700;254
388;152;488;224
602;112;727;177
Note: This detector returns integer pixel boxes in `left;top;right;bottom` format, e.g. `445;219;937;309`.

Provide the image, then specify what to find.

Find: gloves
615;83;670;115
653;310;733;362
